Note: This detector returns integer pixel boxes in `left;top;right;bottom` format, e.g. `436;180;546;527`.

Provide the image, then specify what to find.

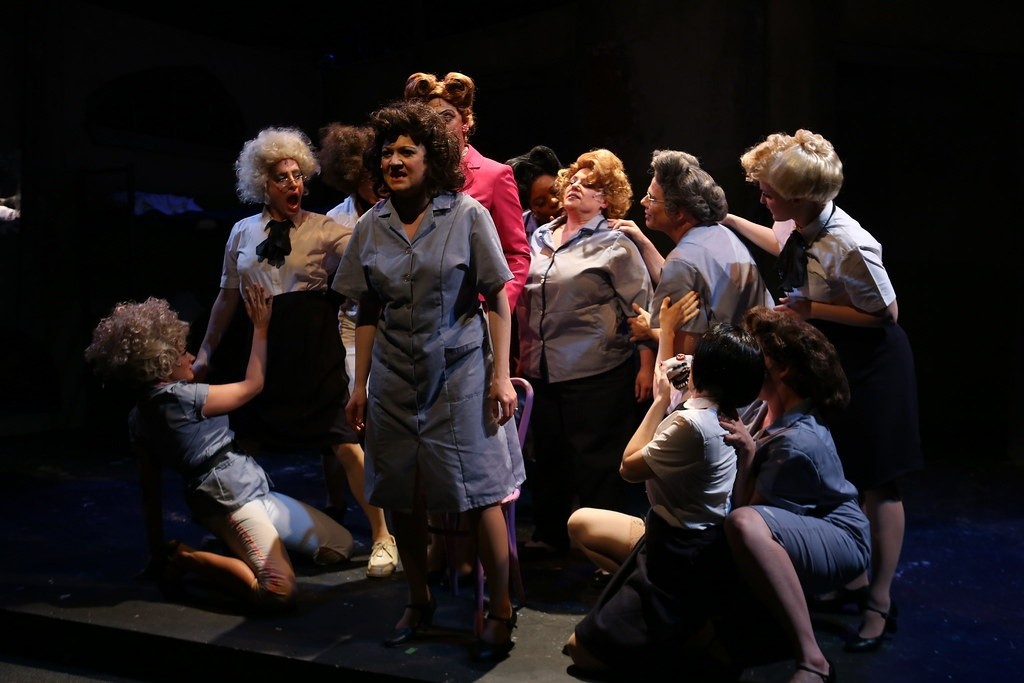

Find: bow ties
776;230;808;291
255;220;295;270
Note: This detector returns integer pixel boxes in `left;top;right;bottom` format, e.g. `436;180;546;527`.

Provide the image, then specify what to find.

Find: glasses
270;175;305;186
646;191;665;203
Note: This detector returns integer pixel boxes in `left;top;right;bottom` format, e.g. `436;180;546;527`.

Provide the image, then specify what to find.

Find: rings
514;408;518;411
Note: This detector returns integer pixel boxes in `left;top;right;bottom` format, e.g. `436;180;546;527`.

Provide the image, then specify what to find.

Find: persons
405;73;530;575
514;150;657;587
189;128;398;578
716;131;917;651
85;284;354;610
717;306;873;683
608;149;775;355
318;123;398;577
567;290;737;683
331;104;526;664
505;146;565;243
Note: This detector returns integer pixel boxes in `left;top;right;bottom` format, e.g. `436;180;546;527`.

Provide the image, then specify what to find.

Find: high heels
812;586;872;607
851;595;897;653
384;600;432;648
469;607;517;663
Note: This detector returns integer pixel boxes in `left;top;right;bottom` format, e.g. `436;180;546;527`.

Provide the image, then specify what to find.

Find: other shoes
137;538;183;591
795;659;837;683
